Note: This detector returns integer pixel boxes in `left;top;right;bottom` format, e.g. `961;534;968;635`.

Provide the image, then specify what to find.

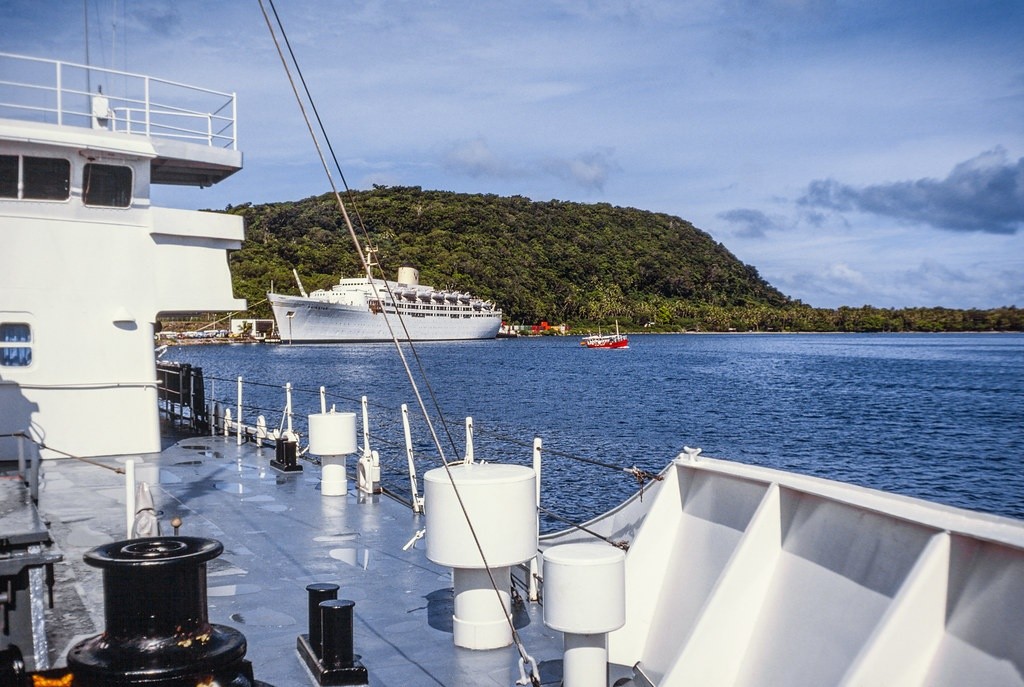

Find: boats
580;319;630;349
265;243;503;344
